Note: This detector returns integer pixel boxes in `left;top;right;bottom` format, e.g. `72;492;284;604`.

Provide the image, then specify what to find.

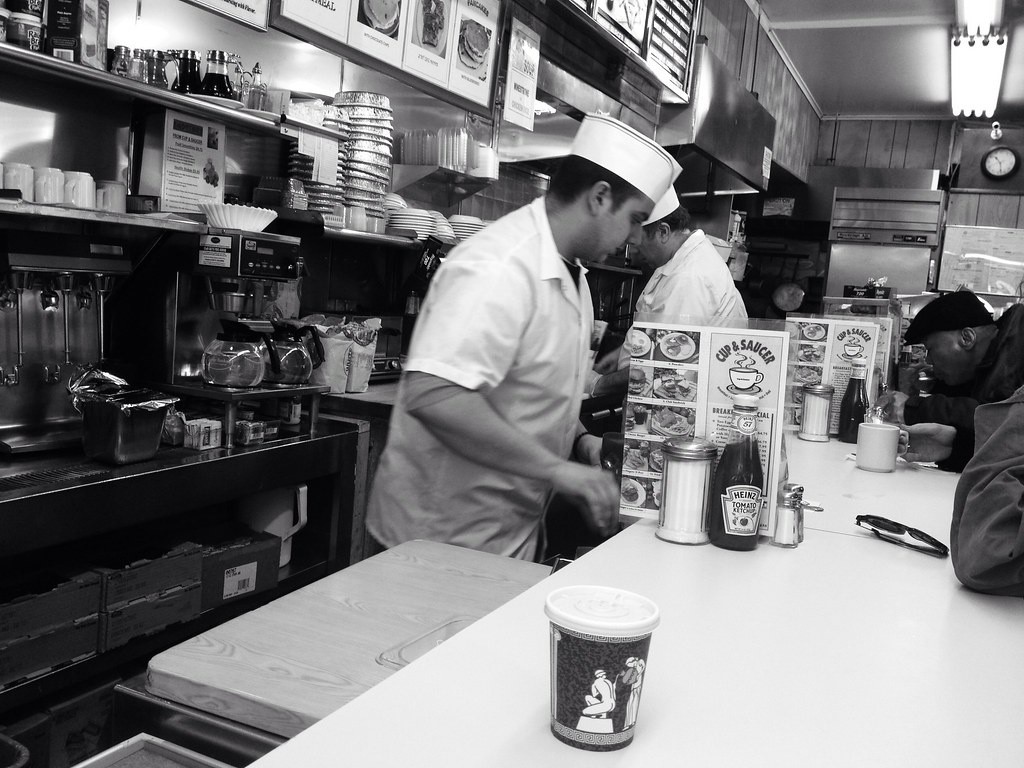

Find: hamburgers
628;369;645;394
660;375;692;397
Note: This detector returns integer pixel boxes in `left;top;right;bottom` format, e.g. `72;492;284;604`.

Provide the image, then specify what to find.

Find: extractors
498;96;582;163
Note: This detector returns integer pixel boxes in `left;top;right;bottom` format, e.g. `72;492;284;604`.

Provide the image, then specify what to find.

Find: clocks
981;144;1021;181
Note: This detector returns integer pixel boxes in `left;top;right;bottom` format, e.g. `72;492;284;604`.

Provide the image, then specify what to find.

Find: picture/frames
180;0;512;119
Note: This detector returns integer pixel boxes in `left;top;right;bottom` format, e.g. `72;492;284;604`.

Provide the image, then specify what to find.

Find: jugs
199;318;282;388
260;318;325;384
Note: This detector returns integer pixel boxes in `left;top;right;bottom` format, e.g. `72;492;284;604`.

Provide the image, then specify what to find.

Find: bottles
837;358;870;444
782;483;804;543
105;44;268;111
654;435;710;546
893;335;912;390
704;394;763;552
798;382;835;442
769;489;798;548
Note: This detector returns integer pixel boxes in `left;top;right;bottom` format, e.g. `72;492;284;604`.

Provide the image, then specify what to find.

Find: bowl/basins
289;89;394;223
402;126;499;181
197;202;278;232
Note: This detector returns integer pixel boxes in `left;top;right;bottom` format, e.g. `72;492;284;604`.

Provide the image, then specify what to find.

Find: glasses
855;515;950;559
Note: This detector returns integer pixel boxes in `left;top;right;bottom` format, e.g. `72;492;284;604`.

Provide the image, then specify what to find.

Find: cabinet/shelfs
0;42;303;262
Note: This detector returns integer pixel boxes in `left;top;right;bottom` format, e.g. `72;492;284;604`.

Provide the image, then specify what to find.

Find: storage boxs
72;387;182;466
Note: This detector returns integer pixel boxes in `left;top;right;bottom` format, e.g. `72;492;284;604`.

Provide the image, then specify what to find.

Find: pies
464;22;490;64
365;0;400;30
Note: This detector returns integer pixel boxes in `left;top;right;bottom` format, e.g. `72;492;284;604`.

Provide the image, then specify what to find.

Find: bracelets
572;432;592;459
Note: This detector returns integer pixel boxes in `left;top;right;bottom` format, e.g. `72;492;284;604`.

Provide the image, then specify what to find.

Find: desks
246;435;1024;768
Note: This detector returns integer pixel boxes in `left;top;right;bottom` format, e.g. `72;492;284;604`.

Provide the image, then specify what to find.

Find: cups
855;422;909;472
897;364;922;407
1;0;46;52
543;584;660;753
0;162;127;214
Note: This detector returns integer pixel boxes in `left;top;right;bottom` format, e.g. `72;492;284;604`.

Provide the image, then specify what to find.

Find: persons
593;178;748;436
886;272;1024;596
365;110;684;566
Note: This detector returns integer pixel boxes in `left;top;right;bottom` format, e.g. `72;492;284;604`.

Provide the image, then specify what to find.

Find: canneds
282;395;302;425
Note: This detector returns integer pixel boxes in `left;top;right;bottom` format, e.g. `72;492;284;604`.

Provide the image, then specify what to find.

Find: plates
382;193;494;241
238;107;282;122
780;318;840;430
185;92;246;110
620;331;696;508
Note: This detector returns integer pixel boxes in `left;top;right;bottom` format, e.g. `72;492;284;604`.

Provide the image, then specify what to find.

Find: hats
897;291;994;345
639;183;680;227
572;111;685;204
